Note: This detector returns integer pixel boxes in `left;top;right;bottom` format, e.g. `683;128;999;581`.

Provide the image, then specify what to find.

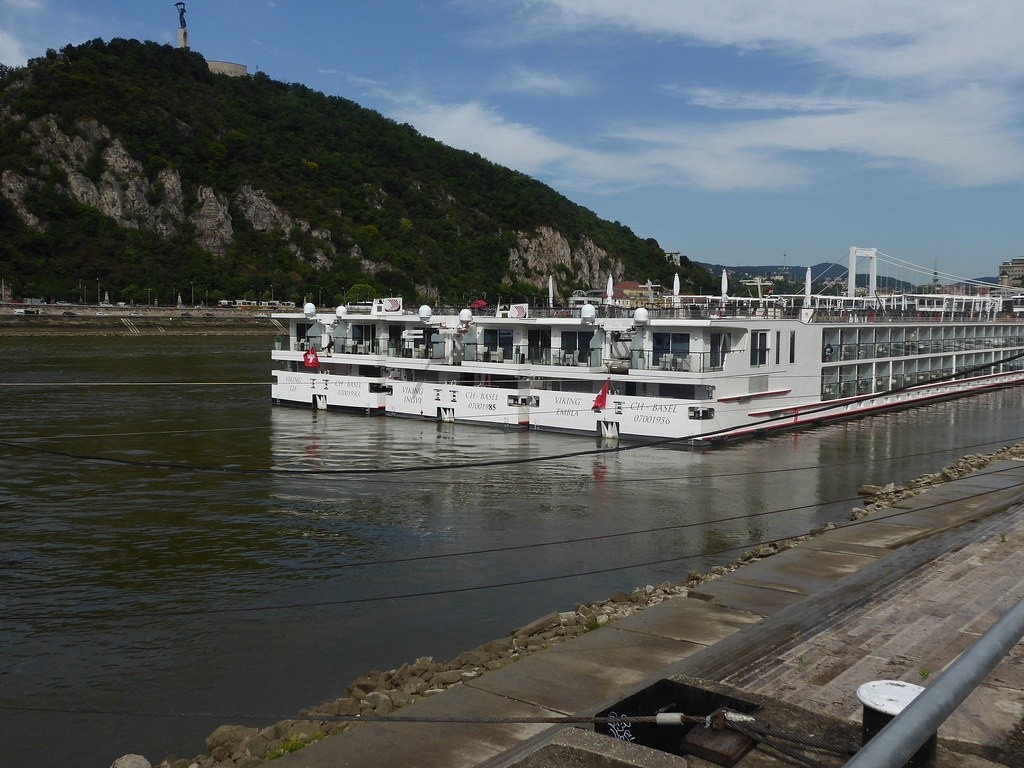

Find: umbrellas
471;300;487;307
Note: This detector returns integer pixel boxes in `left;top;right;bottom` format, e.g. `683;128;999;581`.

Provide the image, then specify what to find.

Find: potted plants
514;346;520;364
275;337;281;349
638;350;644;370
389;342;397;357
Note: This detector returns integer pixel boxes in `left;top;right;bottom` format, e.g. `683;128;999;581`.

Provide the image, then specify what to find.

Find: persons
824;344;833;361
716;308;725;317
325;339;334;353
906;331;916;356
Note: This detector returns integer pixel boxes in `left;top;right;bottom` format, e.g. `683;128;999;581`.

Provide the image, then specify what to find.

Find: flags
303;345;320;369
591;380;609;410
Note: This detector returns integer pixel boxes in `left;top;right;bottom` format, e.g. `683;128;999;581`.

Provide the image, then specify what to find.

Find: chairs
345;341;357;354
677;355;691;372
565;351;580;366
294;339;305;351
659;354;673;370
414;345;426;359
551;350;565;365
357;341;369;355
475;347;489;361
491;348;504;363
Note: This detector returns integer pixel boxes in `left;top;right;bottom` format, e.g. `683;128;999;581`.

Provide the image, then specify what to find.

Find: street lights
9;283;12;303
342;287;345;305
357;293;359;307
390;288;393;299
173;283;177;306
310;292;312;303
319;286;323;308
270;284;274;309
191;281;194;305
96;278;101;306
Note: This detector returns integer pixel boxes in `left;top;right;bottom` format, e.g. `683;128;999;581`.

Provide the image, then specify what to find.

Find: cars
182;312;192;318
203;313;216;318
130;312;143;316
63;312;77;317
95;312;109;316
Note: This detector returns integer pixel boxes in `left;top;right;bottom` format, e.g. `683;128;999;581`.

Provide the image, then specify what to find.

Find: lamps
457;310;472;332
635;308;648;327
336;306;348;319
580;304;596;325
303;303;316;317
419;305;431;321
